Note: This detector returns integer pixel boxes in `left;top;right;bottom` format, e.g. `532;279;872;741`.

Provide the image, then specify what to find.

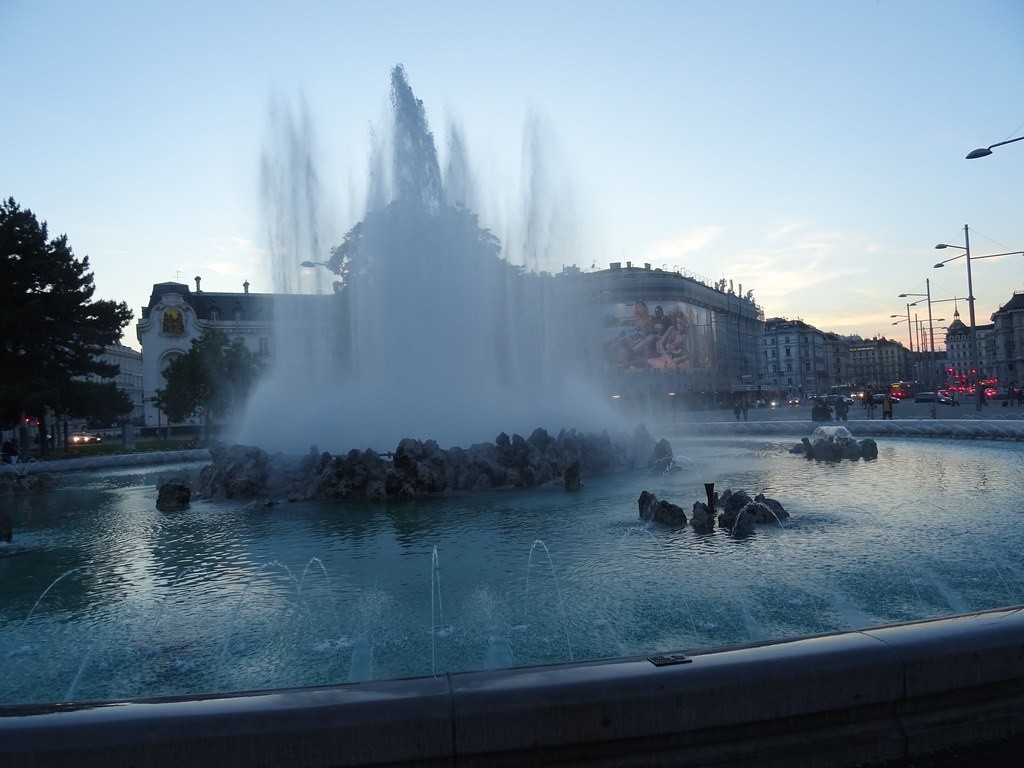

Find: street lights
899;278;938;392
889;302;916;400
932;224;983;413
707;307;725;407
910;317;948;388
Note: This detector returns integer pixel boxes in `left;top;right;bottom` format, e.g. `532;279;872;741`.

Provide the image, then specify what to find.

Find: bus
890;381;913;398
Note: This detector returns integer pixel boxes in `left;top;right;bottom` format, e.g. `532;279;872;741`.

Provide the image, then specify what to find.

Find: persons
732;396;750;423
812;396;849;421
950;390;961;407
977;384;988;409
883;392;893;420
866;390;876;419
1007;385;1024;408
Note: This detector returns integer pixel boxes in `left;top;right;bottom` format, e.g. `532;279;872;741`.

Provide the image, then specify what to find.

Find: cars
789;398;801;405
936;389;953;404
808;384;871;407
68;433;91;444
770;400;781;407
872;393;900;403
